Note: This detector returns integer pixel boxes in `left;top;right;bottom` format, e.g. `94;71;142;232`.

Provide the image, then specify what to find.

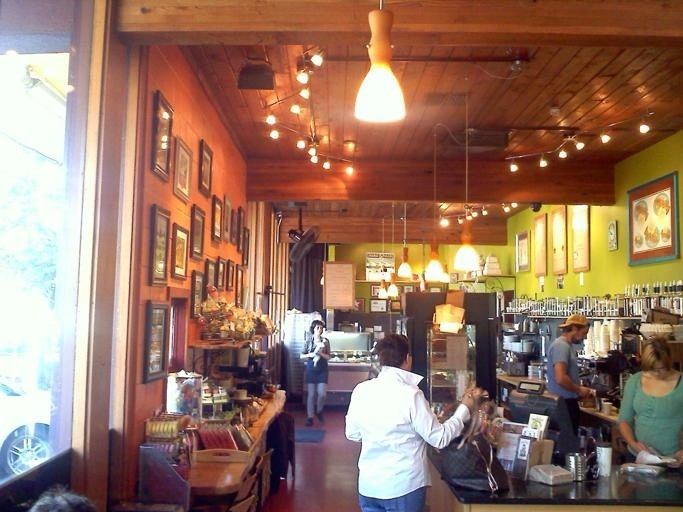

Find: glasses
647;368;667;374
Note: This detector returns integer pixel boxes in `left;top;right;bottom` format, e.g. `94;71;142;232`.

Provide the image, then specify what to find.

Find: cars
0;371;53;479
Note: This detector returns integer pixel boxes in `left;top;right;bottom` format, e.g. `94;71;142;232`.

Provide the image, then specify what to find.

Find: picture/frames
627;171;680;266
322;261;356;310
353;283;442;314
142;89;250;383
516;204;590;277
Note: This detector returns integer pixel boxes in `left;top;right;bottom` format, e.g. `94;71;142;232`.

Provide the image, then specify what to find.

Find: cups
563;452;588;482
602;403;613;417
595;397;610;412
594;442;614;479
586;319;625;359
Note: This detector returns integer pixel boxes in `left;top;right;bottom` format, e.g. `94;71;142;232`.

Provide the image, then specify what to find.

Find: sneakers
304;418;315;427
316;411;324;424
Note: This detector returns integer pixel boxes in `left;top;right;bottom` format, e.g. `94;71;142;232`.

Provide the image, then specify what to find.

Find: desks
146;389;287;512
496;373;619;451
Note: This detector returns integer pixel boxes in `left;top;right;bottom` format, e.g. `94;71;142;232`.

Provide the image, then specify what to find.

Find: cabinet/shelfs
302;361;371;408
425;320;467;409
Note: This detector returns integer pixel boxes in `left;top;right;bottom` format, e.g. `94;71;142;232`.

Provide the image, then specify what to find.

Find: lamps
504;111;658;172
424;122;477;281
259;42;358;176
236;47;275;91
454;56;530;272
378;201;413;299
439;201;519;228
354;0;407;125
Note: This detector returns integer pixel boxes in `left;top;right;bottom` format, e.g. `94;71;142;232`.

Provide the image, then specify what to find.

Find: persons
300;320;330;425
344;335;482;511
616;335;683;472
28;486;99;511
546;314;594;435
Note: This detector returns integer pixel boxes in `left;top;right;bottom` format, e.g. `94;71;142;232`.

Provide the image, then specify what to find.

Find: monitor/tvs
509;390;574;436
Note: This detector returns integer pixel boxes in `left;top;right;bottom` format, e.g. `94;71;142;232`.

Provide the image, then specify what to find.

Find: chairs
192;448;274;512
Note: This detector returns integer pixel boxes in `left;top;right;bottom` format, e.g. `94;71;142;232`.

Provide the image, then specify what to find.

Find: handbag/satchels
440;392;510;495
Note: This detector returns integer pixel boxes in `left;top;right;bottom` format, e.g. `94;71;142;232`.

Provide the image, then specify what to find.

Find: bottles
522;318;548;333
505;279;683;318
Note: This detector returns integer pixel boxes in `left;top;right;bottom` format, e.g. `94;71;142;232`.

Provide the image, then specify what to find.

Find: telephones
529;464;574;486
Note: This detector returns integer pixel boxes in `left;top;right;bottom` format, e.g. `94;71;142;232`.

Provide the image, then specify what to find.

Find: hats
558;313;591;329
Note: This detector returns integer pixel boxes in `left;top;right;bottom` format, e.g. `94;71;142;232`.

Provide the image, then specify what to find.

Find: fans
286;200;320;264
